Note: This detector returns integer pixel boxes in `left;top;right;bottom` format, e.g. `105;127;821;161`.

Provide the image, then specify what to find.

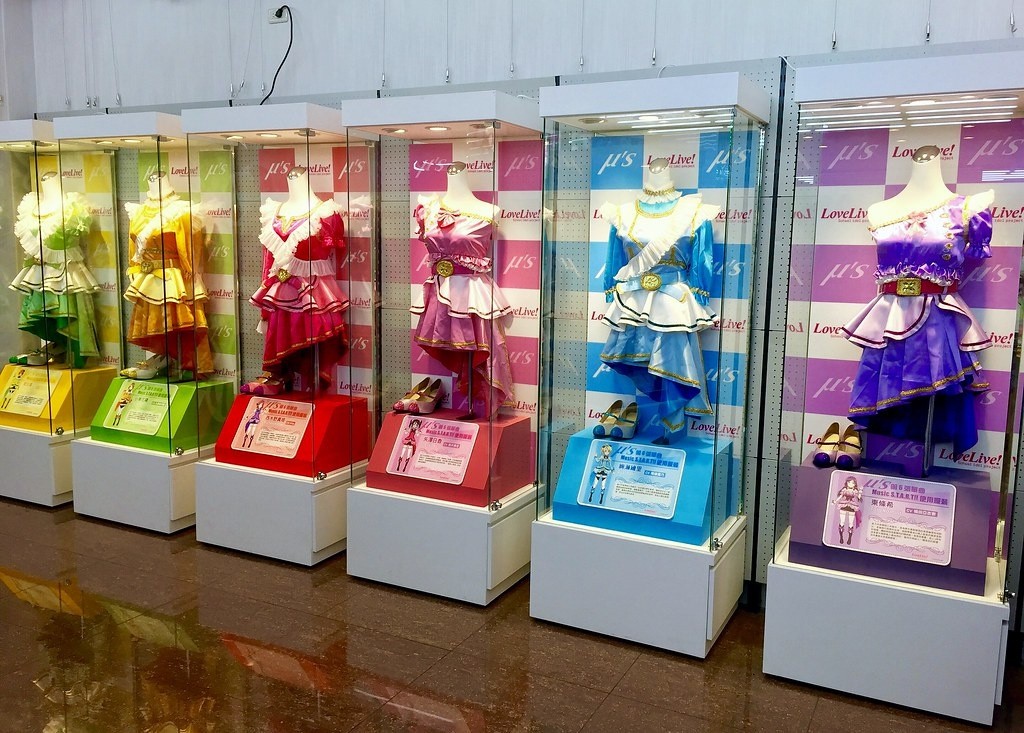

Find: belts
431;261;479;277
878;277;958;294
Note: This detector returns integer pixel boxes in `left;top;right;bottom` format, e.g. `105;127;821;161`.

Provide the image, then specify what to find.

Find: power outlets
268;6;289;25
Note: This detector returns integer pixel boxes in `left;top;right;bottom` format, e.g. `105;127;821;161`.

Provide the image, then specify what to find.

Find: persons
838;146;995;463
599;157;720;435
8;172;104;368
247;167;349;389
415;161;518;419
123;171;215;374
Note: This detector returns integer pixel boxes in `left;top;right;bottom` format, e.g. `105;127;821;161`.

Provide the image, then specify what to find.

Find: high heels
835;425;863;470
408;378;446;414
18;342;66;366
393;377;432;411
593;399;624;436
128;354;173;379
240;372;271;393
253;365;294;396
8;344;49;363
813;422;841;466
119;355;157;376
609;403;640;438
